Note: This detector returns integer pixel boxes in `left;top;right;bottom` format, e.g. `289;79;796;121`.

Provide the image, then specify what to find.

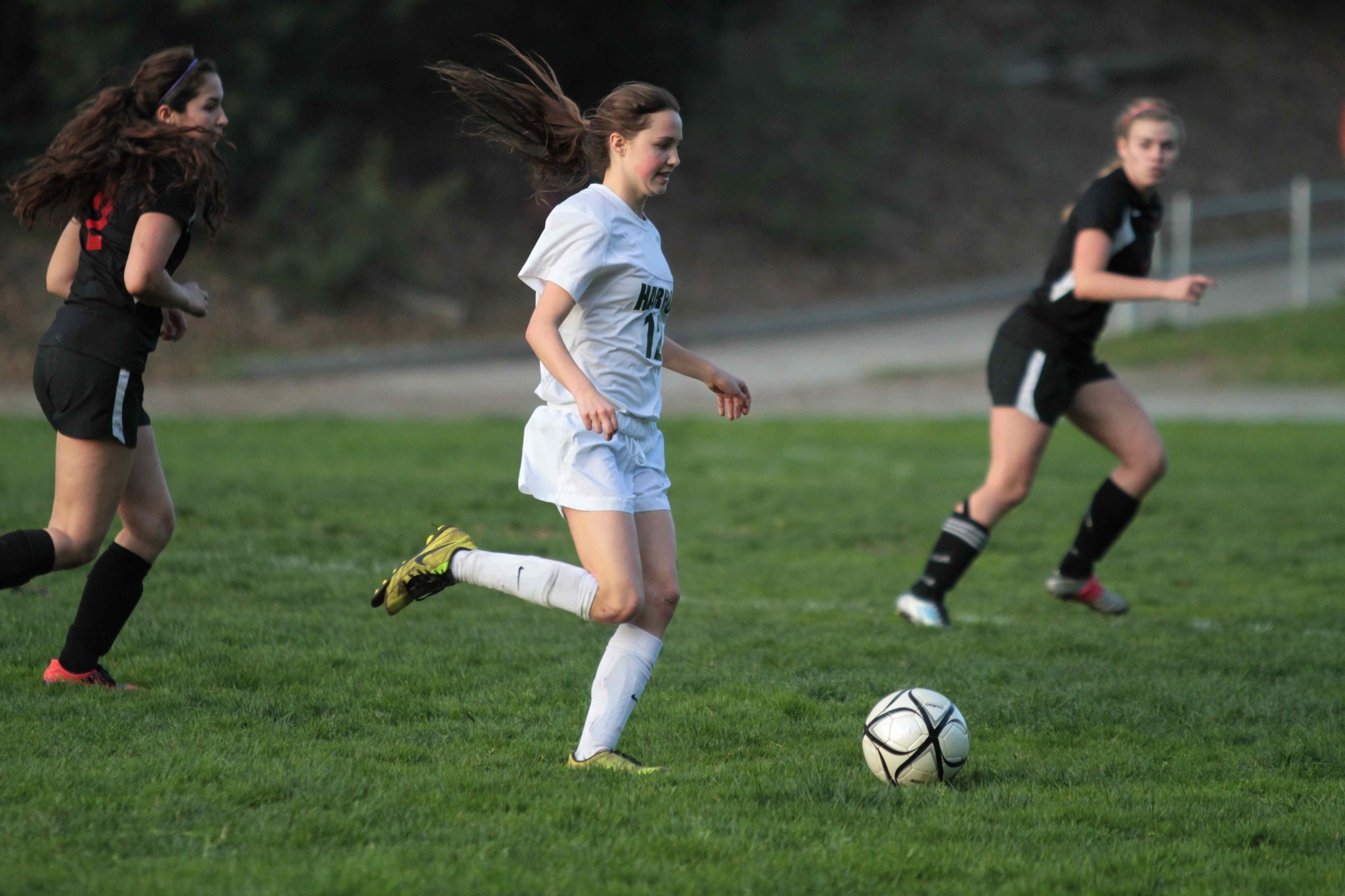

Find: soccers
861;687;970;788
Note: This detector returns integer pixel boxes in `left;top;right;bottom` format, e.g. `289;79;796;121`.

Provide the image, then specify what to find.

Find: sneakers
1046;568;1131;615
42;658;144;691
895;593;952;628
567;748;665;775
370;525;479;616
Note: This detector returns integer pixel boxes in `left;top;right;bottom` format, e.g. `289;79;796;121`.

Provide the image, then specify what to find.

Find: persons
372;28;751;777
0;41;234;691
895;95;1218;628
